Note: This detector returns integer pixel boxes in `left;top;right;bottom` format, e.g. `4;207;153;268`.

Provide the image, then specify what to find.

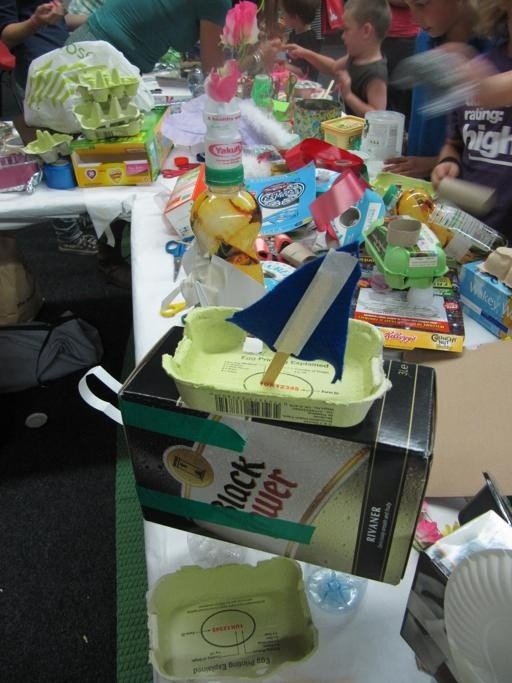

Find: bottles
203;93;242;169
193;165;266;309
251;73;271;105
383;183;508;267
304;564;367;614
188;50;261;96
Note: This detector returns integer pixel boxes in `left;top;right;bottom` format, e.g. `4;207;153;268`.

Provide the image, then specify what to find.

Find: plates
444;548;511;682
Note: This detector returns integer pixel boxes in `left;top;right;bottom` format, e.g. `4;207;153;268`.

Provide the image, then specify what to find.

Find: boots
96;222;131;288
52;222;99;255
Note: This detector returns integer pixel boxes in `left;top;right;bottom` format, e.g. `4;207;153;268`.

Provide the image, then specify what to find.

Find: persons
0;0;512;243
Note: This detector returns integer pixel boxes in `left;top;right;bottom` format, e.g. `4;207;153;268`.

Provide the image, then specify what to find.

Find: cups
293;97;344;141
